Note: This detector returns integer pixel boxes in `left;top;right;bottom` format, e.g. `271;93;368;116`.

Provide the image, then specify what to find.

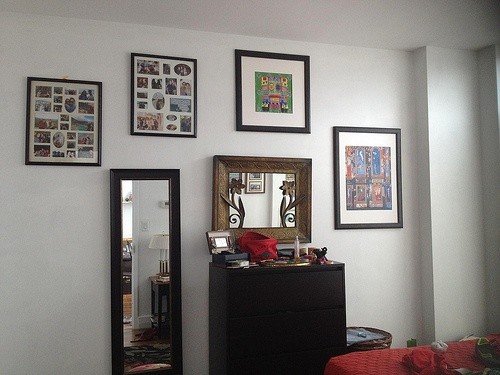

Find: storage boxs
206;229;249;263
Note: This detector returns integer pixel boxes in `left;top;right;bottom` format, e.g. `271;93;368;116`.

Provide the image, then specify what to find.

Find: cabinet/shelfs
209;260;347;375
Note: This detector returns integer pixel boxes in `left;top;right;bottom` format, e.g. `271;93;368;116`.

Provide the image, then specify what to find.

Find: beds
323;334;500;375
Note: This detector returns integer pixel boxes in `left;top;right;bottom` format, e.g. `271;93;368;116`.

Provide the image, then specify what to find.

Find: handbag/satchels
236;231;279;261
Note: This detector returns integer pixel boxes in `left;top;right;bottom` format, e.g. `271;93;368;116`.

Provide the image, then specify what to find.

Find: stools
346;327;393;352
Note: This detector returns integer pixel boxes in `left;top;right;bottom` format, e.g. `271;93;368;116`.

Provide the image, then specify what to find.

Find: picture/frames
228;172;265;194
333;126;404;229
25;76;103;166
130;53;198;138
235;49;311;133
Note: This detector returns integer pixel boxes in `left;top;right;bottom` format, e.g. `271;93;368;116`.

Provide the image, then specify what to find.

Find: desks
149;276;170;338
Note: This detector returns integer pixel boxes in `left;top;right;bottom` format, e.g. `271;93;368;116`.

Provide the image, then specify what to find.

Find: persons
136;58;192;132
34;85;95;160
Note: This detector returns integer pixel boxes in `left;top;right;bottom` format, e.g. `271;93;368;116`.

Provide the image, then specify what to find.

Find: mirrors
212;154;313;244
109;168;183;375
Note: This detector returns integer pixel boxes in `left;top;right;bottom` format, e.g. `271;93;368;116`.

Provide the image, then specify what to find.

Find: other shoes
131;327;157;342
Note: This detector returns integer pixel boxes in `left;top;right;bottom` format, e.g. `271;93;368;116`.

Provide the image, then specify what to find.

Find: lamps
149;234;169;277
